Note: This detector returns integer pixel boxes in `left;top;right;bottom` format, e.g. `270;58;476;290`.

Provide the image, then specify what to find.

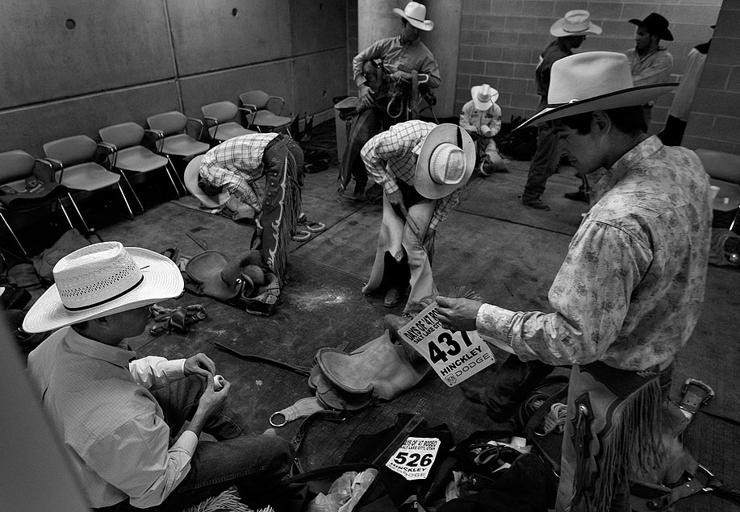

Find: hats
21;240;185;334
393;1;433;32
470;83;499;111
629;12;674;41
510;50;679;133
184;154;232;208
414;122;477;202
549;9;602;38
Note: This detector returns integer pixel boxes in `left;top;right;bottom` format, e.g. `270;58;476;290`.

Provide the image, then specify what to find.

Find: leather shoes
354;177;367;196
461;379;506;424
522;198;548;210
384;289;401;308
565;192;587;201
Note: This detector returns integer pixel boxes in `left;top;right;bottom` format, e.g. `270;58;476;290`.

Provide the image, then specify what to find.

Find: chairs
146;111;211;196
200;100;258;143
693;148;739;248
1;149;73;256
236;89;296;138
98;121;180;215
43;134;134;235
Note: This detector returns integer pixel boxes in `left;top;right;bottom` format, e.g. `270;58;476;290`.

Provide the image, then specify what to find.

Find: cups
710;184;720;201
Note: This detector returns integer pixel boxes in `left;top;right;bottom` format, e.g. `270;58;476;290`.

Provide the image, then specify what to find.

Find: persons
459;84;502;150
666;26;717;146
522;9;603;210
348;1;441;198
565;13;674;203
21;241;294;512
435;51;714;399
184;132;305;309
360;119;477;308
